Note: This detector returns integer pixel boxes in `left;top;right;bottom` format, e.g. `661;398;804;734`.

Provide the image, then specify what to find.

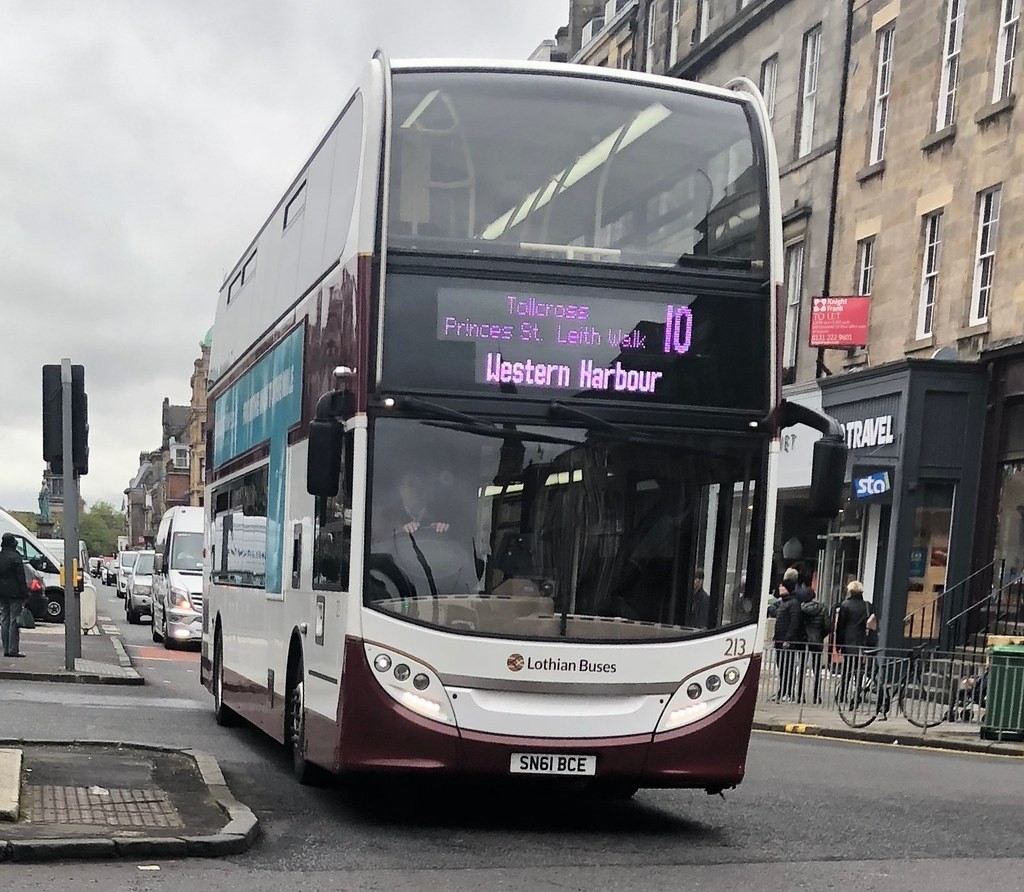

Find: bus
190;46;850;805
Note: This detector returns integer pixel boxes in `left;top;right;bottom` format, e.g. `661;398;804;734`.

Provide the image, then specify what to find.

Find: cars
22;559;51;620
103;560;120;586
89;556;110;585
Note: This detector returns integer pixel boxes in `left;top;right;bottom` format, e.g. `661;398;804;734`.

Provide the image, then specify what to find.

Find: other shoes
797;697;805;704
776;696;795;705
4;652;26;658
812;697;822;704
767;695;780;704
835;695;846;703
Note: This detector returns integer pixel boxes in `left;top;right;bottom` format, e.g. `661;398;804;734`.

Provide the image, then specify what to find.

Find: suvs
123;549;157;624
114;549;138;598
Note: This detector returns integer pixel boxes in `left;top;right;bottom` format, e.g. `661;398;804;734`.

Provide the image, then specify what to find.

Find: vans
34;539;89;573
150;505;205;648
0;508;66;623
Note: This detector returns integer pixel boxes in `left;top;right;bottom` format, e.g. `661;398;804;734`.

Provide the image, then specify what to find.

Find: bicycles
834;642;960;729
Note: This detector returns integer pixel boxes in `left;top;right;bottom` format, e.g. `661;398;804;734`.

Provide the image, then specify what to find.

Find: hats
781;578;795;593
1;533;18;548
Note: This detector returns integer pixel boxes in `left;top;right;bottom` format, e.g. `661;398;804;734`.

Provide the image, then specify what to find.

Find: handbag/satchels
16;602;36;629
865;601;878;655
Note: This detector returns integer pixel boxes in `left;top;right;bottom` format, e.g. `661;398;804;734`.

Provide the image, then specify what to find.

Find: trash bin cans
978;641;1024;741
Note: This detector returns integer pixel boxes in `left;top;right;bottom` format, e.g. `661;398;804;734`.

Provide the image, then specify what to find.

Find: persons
692;563;875;711
93;560;101;579
380;465;484;594
0;533;29;657
